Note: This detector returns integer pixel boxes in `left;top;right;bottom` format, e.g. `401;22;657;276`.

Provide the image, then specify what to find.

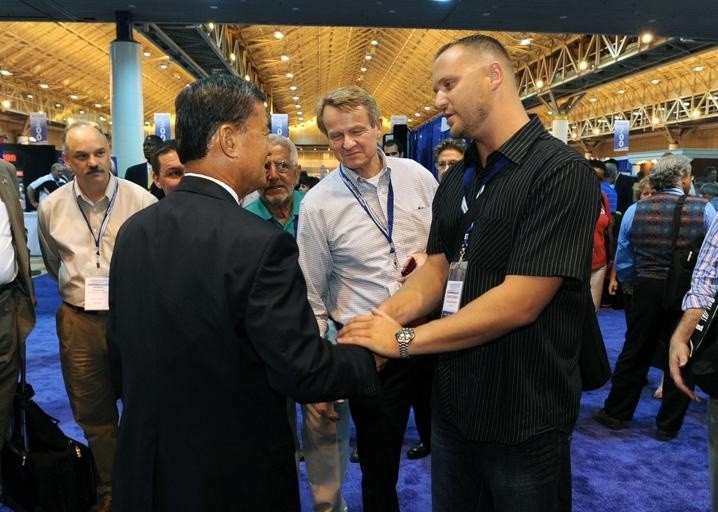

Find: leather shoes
655;429;679;440
592;408;624;430
351;450;359;460
407;440;431;459
90;483;113;512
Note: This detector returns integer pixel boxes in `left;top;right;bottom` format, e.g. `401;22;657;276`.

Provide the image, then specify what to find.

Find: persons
1;86;718;511
335;35;612;512
103;72;391;511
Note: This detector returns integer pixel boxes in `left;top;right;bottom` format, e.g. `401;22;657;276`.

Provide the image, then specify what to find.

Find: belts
63;302;109;317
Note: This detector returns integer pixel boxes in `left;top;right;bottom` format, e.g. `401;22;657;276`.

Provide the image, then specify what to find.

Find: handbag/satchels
6;397;99;511
660;247;702;309
687;298;718;397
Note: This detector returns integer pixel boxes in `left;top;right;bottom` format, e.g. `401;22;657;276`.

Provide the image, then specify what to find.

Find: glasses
264;160;298;170
434;159;458;171
